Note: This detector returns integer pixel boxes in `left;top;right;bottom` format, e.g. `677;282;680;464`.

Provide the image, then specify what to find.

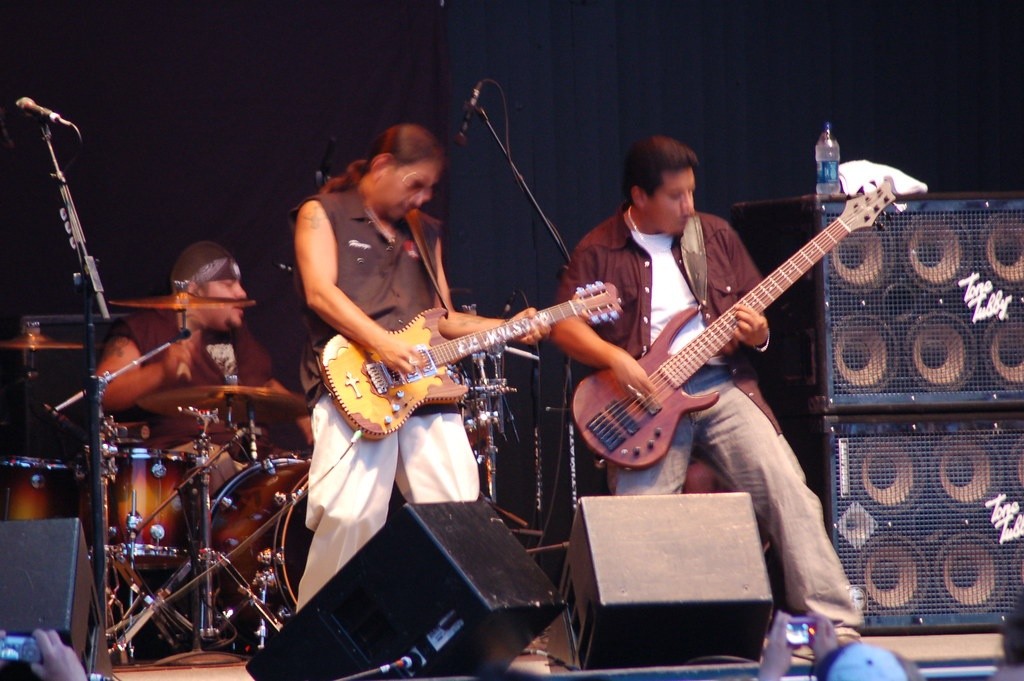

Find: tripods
53;305;284;664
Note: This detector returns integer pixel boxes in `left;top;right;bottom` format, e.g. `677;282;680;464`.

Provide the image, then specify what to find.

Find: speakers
560;492;774;671
734;191;1024;415
0;517;113;679
776;413;1024;639
246;499;567;681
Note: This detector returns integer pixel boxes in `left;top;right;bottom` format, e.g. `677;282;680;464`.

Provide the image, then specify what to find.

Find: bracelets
753;328;770;354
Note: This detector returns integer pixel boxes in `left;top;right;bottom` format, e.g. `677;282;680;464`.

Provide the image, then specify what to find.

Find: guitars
320;279;624;439
571;174;910;471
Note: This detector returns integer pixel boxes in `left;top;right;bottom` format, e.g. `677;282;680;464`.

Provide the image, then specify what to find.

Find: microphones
455;81;483;147
499;291;518;319
39;401;87;441
15;97;74;129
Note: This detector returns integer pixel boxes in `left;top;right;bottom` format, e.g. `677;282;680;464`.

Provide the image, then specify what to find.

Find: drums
207;456;302;522
103;448;195;564
0;456;74;523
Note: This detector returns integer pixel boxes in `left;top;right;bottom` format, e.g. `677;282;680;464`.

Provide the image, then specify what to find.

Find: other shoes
834;624;861;646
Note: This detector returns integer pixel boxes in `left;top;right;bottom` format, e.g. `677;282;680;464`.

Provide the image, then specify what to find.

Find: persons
0;629;88;681
286;119;550;615
96;240;313;520
990;593;1024;681
551;136;866;652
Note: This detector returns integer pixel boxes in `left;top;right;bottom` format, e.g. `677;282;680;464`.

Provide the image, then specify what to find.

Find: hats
816;642;924;681
839;159;929;198
171;241;245;304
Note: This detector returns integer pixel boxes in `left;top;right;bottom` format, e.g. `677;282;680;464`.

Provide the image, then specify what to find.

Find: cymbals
109;290;258;310
5;334;85;351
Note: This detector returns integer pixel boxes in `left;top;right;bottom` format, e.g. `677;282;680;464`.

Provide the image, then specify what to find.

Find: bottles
814;121;841;193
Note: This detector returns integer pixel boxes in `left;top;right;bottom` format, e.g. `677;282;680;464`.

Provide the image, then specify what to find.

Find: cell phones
786;615;816;646
0;630;44;665
